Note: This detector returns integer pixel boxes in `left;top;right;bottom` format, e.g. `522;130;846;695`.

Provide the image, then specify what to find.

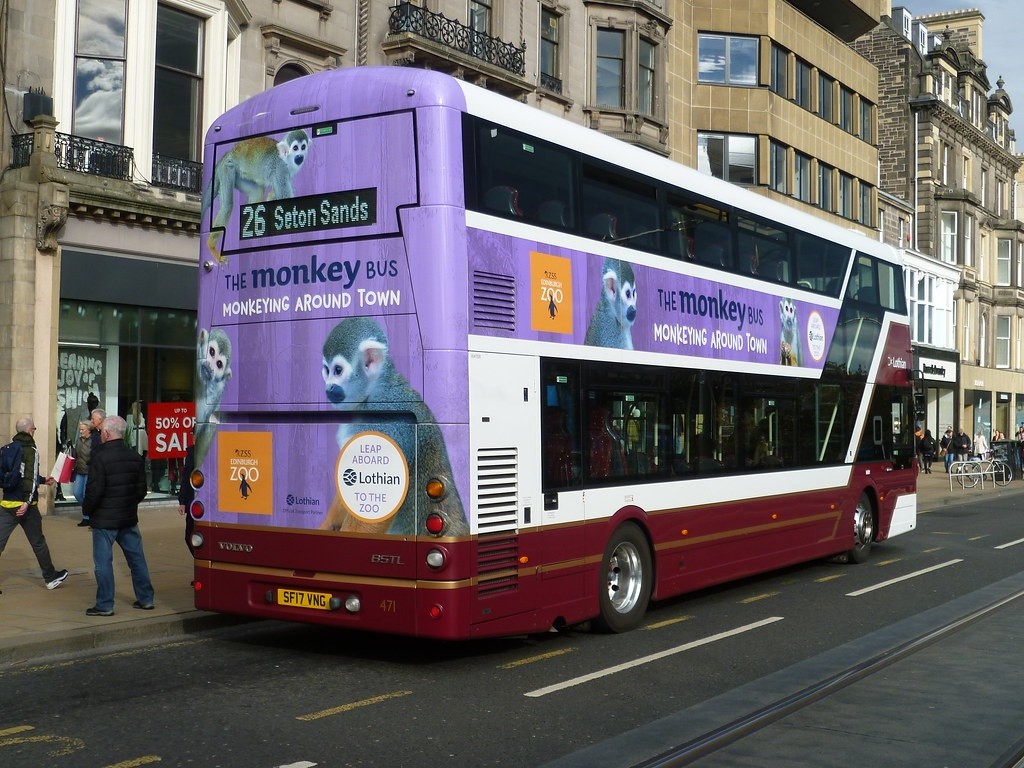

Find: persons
974;429;988;460
915;426;936;474
992;430;1005;441
940;430;962;473
628;409;640;449
692;433;721;470
0;417;69;590
68;409;106;526
178;424;195;586
955;429;971;467
83;416;154;616
759;405;777;442
1015;426;1024;441
750;431;766;446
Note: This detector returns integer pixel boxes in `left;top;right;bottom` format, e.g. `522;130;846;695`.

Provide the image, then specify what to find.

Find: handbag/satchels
940;450;946;456
51;445;76;483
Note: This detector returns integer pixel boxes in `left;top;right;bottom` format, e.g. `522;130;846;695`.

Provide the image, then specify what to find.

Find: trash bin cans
989;439;1023;481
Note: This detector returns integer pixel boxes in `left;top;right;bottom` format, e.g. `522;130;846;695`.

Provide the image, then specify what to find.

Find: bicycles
956;449;1013;488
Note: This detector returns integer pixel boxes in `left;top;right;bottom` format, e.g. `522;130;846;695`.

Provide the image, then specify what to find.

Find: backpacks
922;436;930;451
0;443;24;492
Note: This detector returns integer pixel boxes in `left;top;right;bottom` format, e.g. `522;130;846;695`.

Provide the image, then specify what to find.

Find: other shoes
78;519;89;526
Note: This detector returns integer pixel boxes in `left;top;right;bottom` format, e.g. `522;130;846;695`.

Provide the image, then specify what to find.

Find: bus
188;64;926;642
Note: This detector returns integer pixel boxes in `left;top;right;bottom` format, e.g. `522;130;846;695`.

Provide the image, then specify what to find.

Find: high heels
55;493;66;501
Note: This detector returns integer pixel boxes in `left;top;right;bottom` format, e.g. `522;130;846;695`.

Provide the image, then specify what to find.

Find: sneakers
133;600;153;609
45;569;69;589
86;607;114;616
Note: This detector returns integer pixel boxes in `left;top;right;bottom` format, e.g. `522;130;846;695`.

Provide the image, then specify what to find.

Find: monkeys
316;317;471;538
196;327;233;467
200;129;313;271
779;295;803;366
584;256;638;351
